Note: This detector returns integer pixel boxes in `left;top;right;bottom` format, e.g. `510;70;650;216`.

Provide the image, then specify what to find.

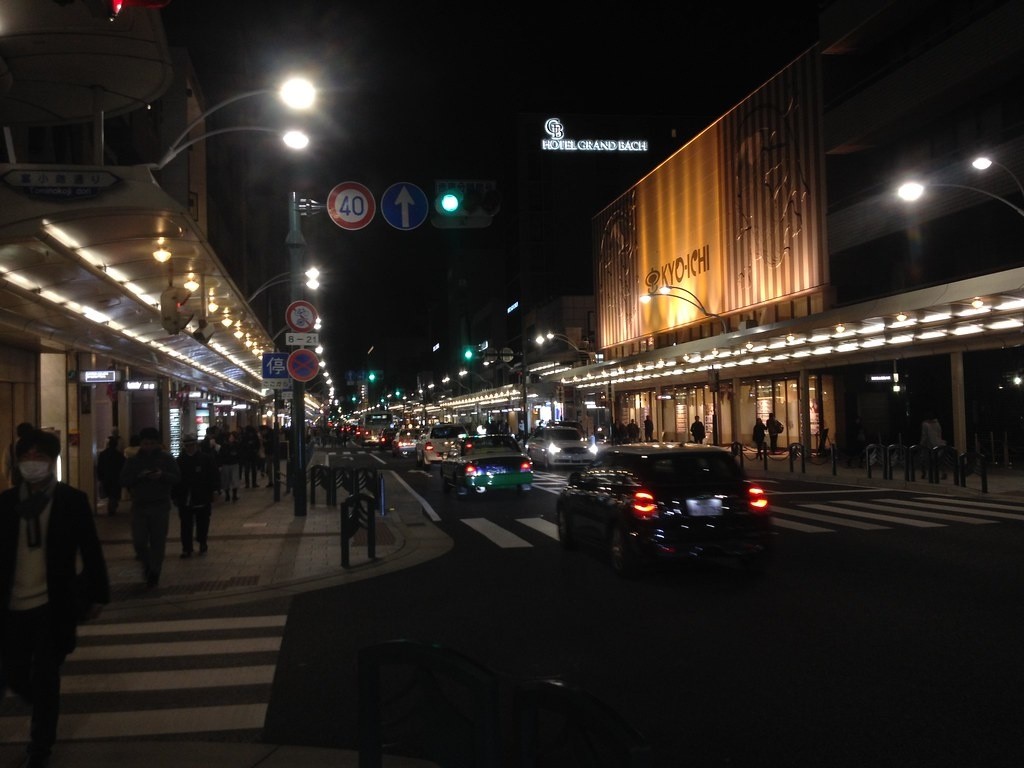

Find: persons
96;426;292;595
0;422;110;768
518;420;525;436
691;415;705;444
767;413;778;451
644;415;653;442
613;419;639;445
752;418;767;453
919;408;948;480
535;421;544;434
306;423;357;449
484;419;510;435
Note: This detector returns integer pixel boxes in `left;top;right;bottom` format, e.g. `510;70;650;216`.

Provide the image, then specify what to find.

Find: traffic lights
427;178;501;226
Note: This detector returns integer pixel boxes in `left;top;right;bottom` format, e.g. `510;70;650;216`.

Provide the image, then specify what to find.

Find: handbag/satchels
58;569;93;625
772;421;783;433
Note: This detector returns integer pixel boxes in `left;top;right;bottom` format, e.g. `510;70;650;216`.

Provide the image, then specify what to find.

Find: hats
182;433;197;445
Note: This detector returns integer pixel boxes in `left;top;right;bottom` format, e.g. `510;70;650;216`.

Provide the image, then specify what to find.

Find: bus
357;413;394;449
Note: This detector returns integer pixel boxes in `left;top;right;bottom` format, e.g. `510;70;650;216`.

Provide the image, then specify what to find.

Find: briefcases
99;479;107;499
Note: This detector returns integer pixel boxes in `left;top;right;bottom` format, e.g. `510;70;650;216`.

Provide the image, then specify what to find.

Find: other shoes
140;569;159;590
24;752;52;768
180;545;209;559
243;482;273;489
224;496;239;502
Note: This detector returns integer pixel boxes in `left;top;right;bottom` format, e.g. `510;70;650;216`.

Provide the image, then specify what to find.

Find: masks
15;454;54;486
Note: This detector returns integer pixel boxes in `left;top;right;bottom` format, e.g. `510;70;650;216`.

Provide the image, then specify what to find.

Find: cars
441;431;534;498
391;429;421;456
415;425;469;468
557;441;774;583
515;424;590;465
379;427;404;448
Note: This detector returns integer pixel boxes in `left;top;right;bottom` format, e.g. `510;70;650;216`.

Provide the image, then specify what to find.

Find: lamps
897;156;1024;216
639;285;728;334
154;76;315;171
536;332;589;354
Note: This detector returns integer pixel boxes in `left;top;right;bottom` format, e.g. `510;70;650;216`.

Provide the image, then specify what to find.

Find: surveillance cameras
193;323;215;341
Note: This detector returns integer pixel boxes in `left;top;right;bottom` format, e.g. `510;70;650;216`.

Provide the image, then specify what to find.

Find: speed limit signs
325;179;376;231
